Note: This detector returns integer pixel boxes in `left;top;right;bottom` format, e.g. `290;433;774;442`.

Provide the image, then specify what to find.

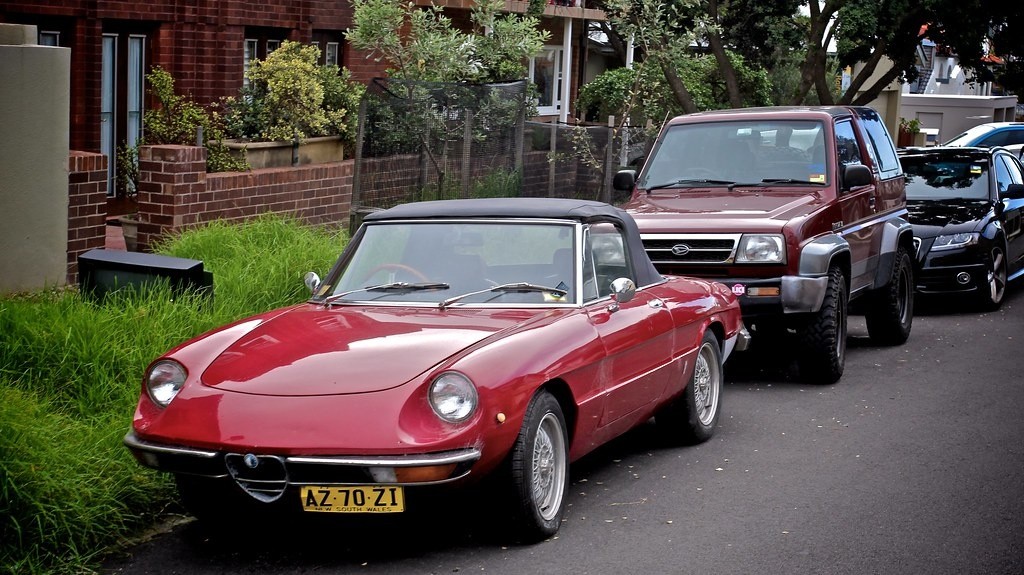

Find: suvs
586;105;917;383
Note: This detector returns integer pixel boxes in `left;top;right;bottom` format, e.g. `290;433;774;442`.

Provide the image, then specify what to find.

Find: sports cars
121;196;742;542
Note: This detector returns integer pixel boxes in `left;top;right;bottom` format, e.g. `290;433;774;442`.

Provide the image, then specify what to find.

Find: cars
931;120;1023;148
894;144;1024;311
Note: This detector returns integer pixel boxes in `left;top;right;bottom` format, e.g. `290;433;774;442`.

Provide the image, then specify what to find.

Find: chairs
440;248;613;305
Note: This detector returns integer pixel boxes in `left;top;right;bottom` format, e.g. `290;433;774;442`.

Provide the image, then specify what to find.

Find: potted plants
208;39;358;172
898;117;921;149
